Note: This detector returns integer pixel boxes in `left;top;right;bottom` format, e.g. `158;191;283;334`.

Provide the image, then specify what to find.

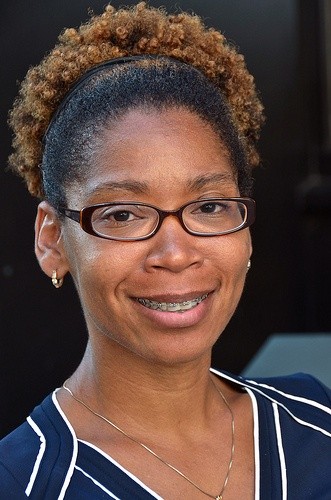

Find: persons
1;0;331;499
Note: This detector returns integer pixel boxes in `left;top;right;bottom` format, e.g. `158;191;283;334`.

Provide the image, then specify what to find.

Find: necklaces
63;377;236;499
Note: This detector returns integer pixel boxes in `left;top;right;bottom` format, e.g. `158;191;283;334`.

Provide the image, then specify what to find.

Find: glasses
59;197;256;242
